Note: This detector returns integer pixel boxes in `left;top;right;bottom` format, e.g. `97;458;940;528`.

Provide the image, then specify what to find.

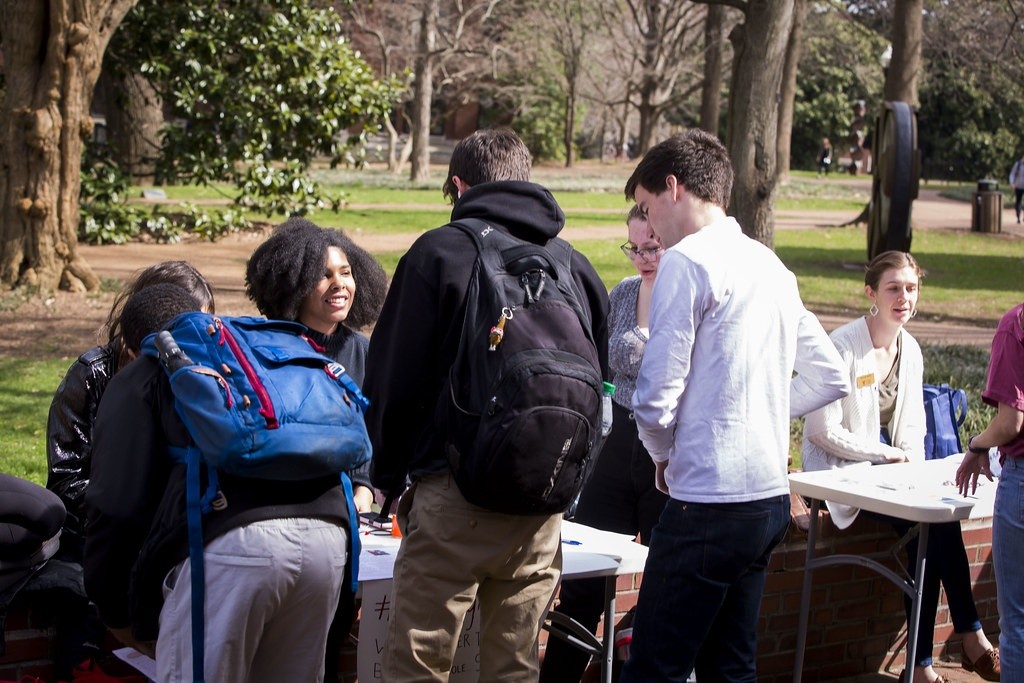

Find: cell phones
359;511;393;529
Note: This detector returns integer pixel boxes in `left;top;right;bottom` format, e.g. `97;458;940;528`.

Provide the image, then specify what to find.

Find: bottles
600;382;616;435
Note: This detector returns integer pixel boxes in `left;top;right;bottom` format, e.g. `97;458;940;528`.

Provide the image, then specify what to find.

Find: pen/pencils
878;484;897;492
561;539;582;546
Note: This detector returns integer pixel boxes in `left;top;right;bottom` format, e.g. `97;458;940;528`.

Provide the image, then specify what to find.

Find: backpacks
144;311;376;481
439;218;603;518
612;606;696;682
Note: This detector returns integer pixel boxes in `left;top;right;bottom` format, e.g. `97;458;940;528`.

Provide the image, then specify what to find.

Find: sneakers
899;669;953;683
960;641;1000;682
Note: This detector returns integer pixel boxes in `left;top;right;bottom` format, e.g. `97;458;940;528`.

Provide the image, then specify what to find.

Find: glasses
621;241;662;263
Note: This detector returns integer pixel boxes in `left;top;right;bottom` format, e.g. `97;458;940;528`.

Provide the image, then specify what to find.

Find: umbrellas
153;330;192;374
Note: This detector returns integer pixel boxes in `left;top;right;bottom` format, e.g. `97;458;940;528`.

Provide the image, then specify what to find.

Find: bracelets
968;435;990;453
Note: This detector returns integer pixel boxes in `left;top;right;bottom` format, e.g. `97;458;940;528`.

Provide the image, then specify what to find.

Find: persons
801;250;1000;683
86;285;359;683
46;262;215;563
619;131;851;683
816;138;832;176
364;127;612;683
1009;158;1024;224
537;207;669;683
245;218;387;510
955;304;1024;683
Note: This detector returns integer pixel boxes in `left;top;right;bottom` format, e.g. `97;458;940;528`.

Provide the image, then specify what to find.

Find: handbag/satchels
924;381;967;460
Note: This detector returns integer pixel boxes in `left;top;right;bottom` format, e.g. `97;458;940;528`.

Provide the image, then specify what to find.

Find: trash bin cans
973;181;1002;234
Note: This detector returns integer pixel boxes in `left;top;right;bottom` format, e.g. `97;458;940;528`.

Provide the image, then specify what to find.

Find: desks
787;452;999;683
359;519;651;683
